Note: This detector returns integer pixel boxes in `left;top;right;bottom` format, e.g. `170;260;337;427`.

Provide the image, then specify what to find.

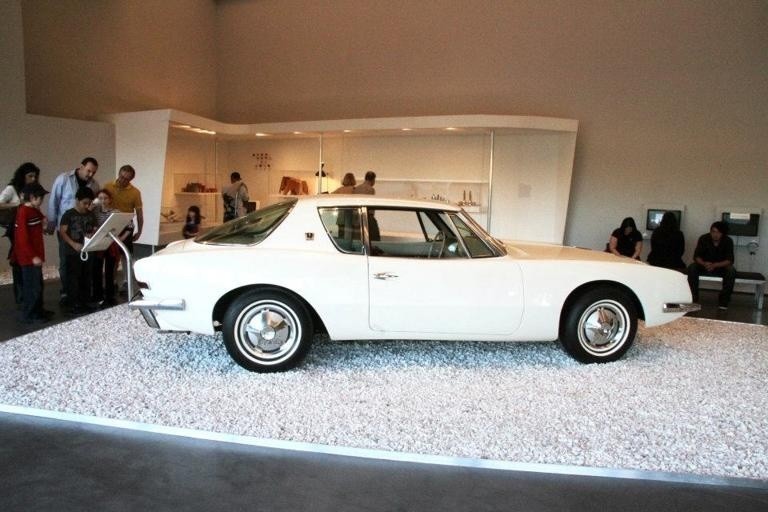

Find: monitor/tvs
723;212;760;236
646;209;681;230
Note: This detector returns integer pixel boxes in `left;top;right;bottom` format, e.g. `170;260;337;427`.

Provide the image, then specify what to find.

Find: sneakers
719;302;729;310
59;281;127;314
22;309;54;323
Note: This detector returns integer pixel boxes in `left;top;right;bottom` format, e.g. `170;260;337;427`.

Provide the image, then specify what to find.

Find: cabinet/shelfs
271;178;488;214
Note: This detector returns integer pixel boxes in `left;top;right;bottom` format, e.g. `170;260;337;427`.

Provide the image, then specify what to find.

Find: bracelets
633;254;636;258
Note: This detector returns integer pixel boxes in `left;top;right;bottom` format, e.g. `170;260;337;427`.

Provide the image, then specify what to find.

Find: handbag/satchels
224;193;237;222
0;207;17;227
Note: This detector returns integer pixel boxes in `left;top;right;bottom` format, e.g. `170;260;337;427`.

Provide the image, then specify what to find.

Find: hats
24;183;49;196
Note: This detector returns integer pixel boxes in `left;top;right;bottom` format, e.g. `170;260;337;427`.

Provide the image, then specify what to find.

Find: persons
103;165;143;309
222;169;249;223
644;210;688;274
332;173;361;239
182;205;201;240
605;216;643;262
353;170;377;248
14;182;56;324
0;162;41;303
48;157;101;306
92;188;132;307
688;219;737;313
60;186;98;312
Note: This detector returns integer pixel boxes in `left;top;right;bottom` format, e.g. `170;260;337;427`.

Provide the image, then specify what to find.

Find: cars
130;194;702;372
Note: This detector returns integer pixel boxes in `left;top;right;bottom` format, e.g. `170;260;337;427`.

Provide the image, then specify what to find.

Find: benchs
699;272;766;310
